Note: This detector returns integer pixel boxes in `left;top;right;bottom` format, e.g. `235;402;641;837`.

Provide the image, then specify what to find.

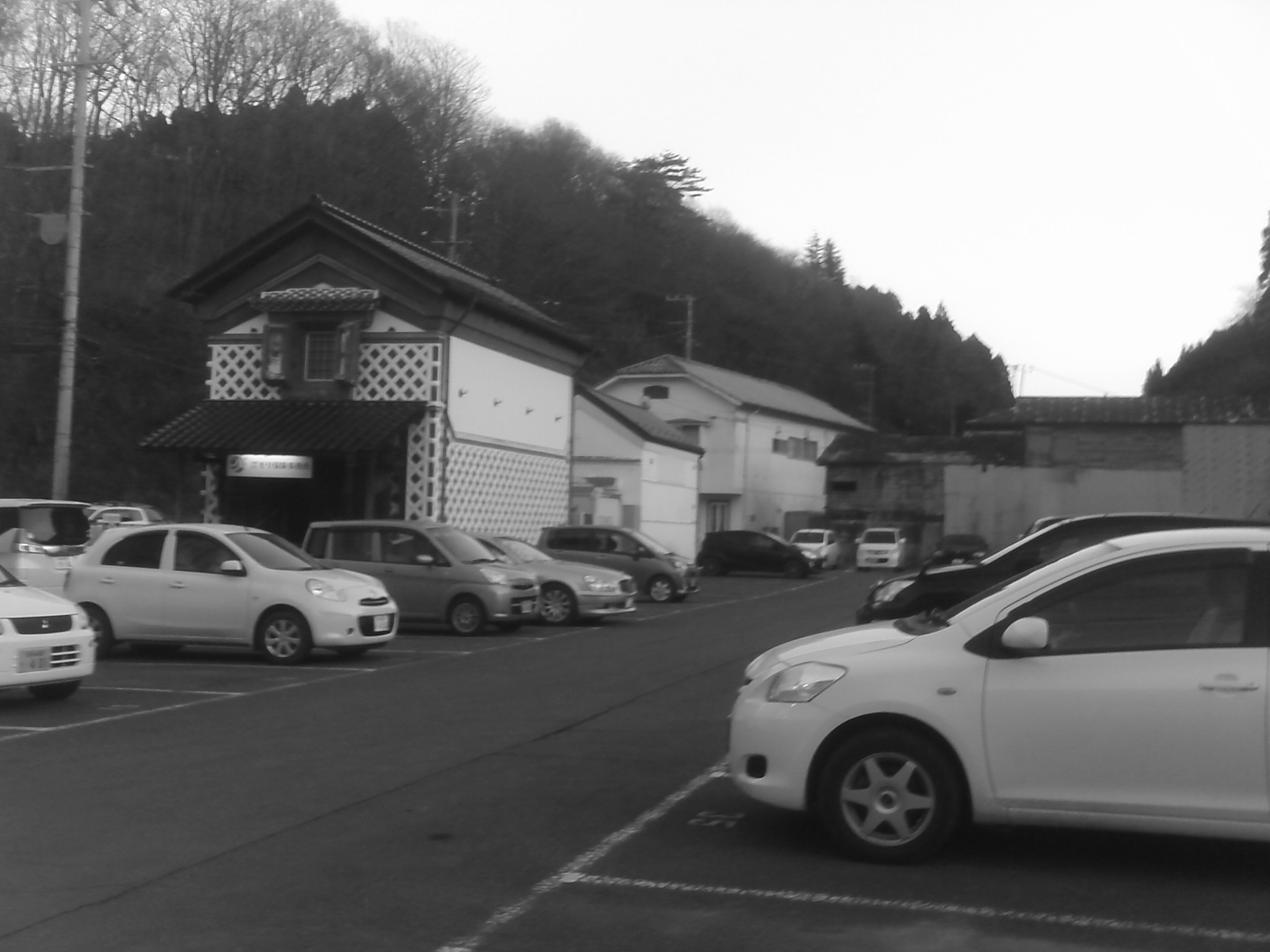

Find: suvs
852;512;1270;629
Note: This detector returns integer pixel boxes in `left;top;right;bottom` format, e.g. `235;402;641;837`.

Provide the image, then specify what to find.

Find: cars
721;526;1270;863
788;529;844;572
0;562;97;702
486;535;638;628
929;532;987;568
69;523;402;665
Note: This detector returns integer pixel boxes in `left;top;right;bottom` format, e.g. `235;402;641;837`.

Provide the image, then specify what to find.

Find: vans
303;518;544;635
855;526;921;571
0;497;172;603
694;529;826;579
537;523;700;602
